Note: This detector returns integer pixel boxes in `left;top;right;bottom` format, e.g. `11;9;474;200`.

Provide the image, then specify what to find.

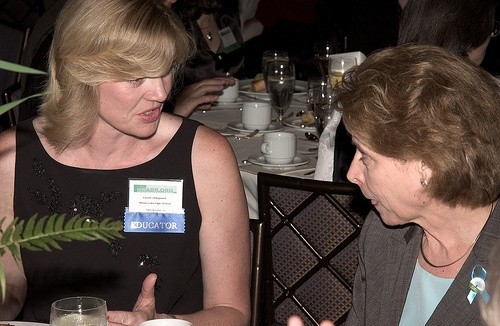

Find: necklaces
420;202;494;268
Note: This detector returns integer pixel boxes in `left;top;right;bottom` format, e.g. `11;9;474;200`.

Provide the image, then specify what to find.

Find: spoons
305;132;319;142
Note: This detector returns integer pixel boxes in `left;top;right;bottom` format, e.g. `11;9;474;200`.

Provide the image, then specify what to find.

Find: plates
212;100;248;109
238;79;308;100
281;116;324;131
227;121;285;133
248;155;311;169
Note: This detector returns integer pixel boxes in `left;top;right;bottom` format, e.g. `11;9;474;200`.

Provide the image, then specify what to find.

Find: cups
210;77;240;103
140;318;192;326
50;296;108;326
261;132;297;164
240;103;272;130
328;56;357;94
262;51;289;92
313;81;338;132
315;40;340;76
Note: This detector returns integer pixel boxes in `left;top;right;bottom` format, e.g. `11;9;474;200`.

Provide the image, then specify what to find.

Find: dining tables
188;78;329;221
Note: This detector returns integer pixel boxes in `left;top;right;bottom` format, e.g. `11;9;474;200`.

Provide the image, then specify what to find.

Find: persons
332;0;500;186
18;1;235;117
287;44;500;326
0;0;253;326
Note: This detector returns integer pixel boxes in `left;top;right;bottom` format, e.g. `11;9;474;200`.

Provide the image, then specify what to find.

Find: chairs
248;219;267;326
0;15;43;133
256;171;373;326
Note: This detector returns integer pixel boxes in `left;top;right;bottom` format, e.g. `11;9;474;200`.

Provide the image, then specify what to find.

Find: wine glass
267;62;295;126
